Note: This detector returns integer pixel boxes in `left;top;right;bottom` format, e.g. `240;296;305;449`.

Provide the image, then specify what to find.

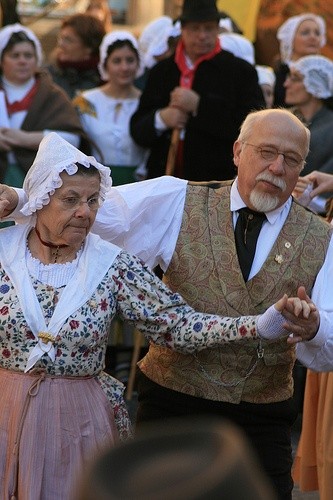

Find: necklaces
35;224;70;264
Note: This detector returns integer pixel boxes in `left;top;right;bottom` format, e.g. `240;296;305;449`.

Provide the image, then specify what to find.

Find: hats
173;0;226;26
218;33;255;65
19;132;112;216
77;419;275;500
255;65;276;87
295;55;333;99
137;17;181;70
277;14;326;65
219;18;233;33
0;22;41;71
97;32;145;82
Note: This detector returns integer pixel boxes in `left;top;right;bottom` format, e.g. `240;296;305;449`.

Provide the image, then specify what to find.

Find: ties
235;208;267;284
0;79;39;116
175;37;221;90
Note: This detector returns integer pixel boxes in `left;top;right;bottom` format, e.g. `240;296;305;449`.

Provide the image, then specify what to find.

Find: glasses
55;192;103;212
286;72;302;82
242;143;302;167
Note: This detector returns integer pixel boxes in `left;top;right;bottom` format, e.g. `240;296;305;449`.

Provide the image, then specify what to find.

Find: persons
0;132;315;500
0;0;333;382
0;110;333;500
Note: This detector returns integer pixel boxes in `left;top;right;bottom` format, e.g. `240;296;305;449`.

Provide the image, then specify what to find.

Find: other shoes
115;351;138;384
